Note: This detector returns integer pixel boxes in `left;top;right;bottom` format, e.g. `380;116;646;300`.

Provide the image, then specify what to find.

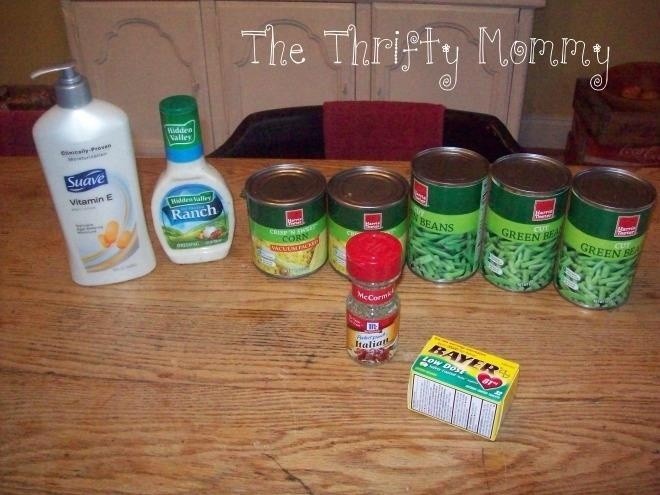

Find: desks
2;152;658;490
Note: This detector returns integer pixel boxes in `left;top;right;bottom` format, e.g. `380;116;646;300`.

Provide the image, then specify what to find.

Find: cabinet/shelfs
66;0;545;168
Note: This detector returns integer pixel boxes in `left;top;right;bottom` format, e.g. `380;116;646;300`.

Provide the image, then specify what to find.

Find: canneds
406;147;491;284
243;164;329;279
327;167;410;280
554;166;657;310
478;152;574;294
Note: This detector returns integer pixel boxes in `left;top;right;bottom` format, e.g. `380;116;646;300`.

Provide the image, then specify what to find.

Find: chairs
199;103;522;173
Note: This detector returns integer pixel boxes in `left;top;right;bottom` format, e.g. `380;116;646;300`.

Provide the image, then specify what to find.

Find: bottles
148;94;236;265
343;230;404;365
